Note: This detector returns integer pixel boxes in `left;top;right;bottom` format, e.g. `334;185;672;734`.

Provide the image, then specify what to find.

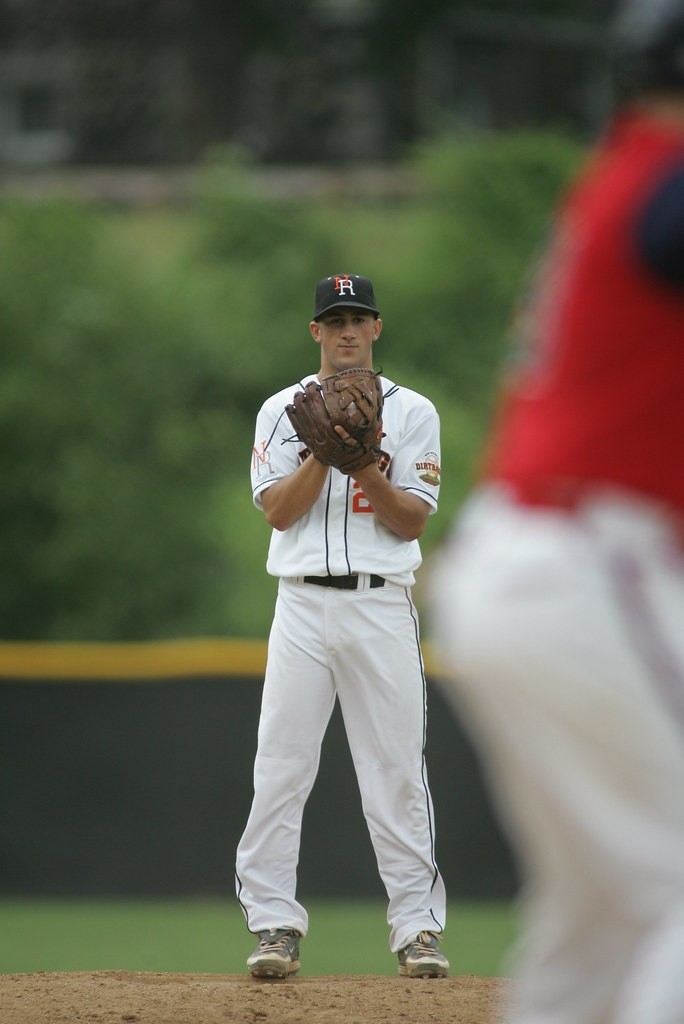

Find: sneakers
246;928;300;977
398;931;449;979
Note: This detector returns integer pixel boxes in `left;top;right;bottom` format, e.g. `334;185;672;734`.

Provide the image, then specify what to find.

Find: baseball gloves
284;365;385;476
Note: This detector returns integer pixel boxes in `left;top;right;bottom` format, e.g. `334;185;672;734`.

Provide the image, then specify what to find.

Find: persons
431;13;684;1024
233;274;459;976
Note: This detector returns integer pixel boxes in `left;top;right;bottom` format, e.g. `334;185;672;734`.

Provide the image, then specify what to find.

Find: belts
304;574;385;589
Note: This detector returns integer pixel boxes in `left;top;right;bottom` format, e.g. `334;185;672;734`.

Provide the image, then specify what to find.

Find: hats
313;274;380;322
